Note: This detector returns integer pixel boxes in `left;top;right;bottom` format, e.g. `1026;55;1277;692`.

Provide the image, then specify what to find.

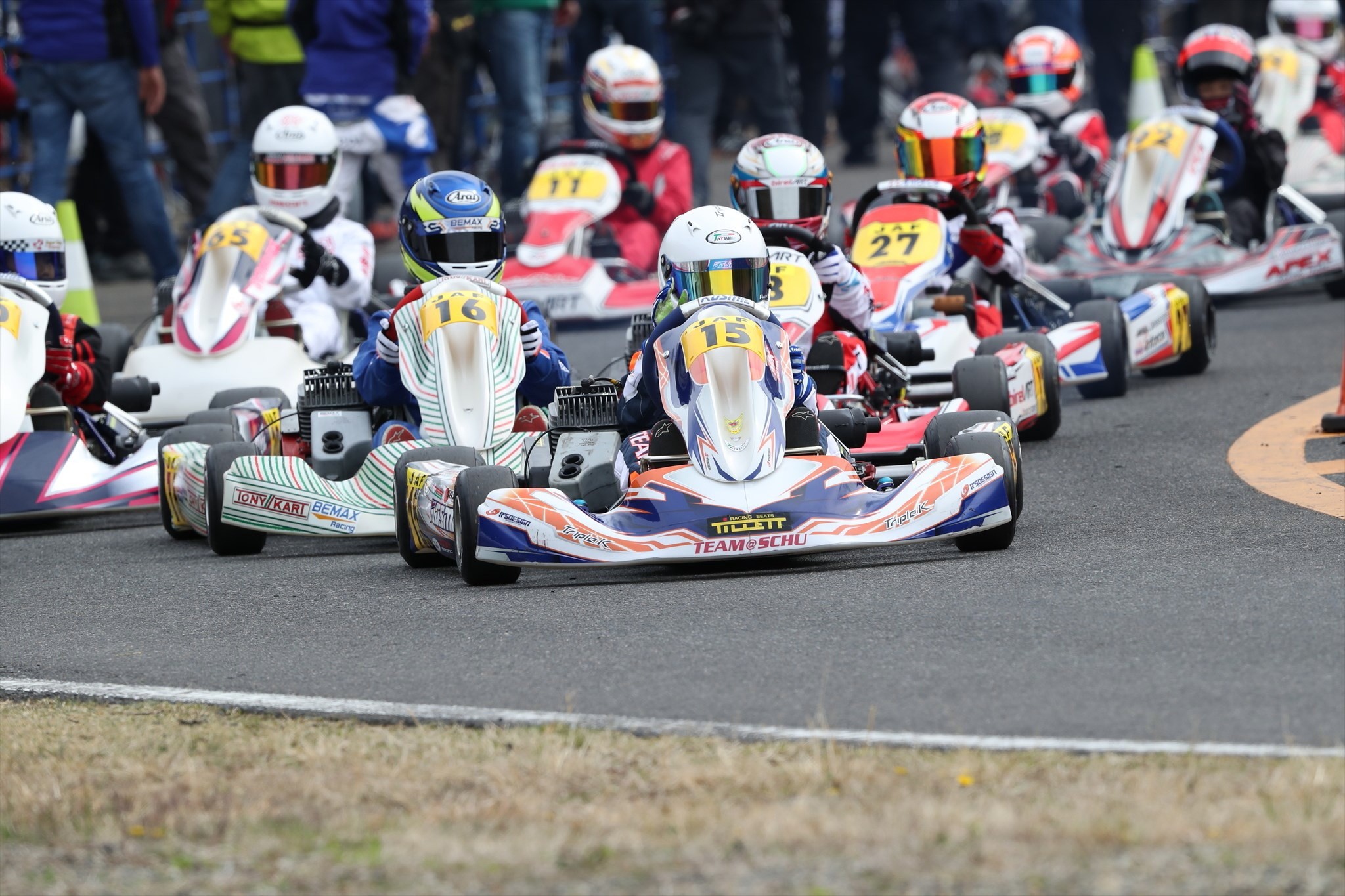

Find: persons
153;106;376;363
843;93;1025;339
353;169;572;446
0;191;112;443
0;0;1345;286
729;134;875;410
613;206;848;500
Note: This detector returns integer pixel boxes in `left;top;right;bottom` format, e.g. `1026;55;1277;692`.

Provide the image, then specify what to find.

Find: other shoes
265;301;302;343
512;404;548;432
1193;191;1228;236
160;302;176;344
784;407;820;458
944;278;977;333
29;384;72;433
590;226;622;280
648;419;688;470
382;423;416;446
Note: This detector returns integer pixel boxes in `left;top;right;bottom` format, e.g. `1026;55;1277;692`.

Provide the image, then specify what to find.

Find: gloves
623;176;654;215
957;215;1006;266
519;320;543;359
46;336;84;392
788;346;812;403
375;317;401;364
1226;82;1261;137
812;244;858;289
288;236;351;287
1049;130;1095;177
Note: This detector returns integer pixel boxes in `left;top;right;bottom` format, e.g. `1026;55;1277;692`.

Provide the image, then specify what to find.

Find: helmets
1006;25;1087;123
730;133;833;253
0;191;69;308
893;91;987;194
398;170;507;289
1267;0;1345;63
581;44;666;150
1177;24;1263;122
658;205;772;318
248;105;340;219
